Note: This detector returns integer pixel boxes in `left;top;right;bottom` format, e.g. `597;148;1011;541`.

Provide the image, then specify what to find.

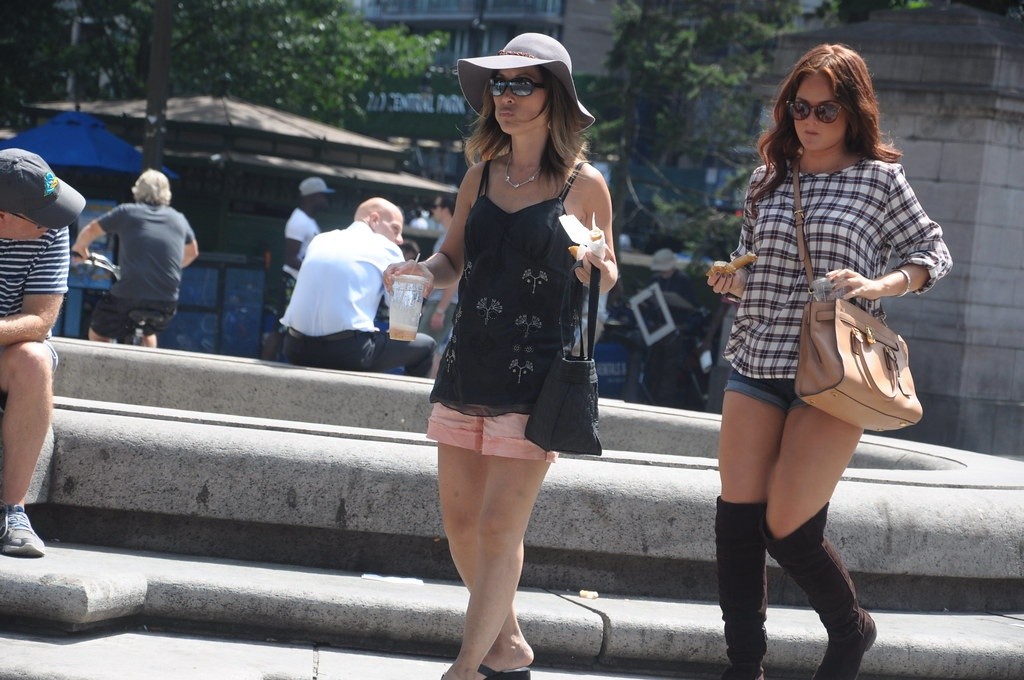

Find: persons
702;290;741;415
380;33;619;680
71;165;200;350
280;196;436;377
0;147;87;556
648;250;703;333
263;175;335;363
421;194;461;380
376;237;423;375
703;43;957;680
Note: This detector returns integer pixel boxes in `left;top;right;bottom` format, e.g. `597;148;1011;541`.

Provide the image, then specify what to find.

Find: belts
287;327;362;342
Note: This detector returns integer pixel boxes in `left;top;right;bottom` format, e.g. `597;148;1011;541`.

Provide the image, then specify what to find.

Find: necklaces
505;155;542;189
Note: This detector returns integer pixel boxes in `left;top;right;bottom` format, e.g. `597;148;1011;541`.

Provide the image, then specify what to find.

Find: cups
809;278;849;301
389;274;427;342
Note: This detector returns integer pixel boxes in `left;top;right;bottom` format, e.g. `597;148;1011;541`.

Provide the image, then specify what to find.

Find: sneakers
0;500;45;559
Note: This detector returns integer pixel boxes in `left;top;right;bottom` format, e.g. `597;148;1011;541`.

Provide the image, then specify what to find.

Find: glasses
486;74;548;98
10;212;43;229
783;100;846;123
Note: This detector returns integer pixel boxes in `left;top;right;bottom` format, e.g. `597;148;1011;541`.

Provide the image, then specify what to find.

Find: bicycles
70;248;188;345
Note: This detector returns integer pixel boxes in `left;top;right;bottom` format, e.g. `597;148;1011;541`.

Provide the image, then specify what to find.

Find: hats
457;33;595;134
0;148;87;229
298;177;336;196
650;248;678;272
427;197;454;209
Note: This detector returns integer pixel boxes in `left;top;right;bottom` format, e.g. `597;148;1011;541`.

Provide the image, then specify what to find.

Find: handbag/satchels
795;296;923;431
525;258;603;455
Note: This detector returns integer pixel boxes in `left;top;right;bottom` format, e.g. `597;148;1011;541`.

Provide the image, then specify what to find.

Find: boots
715;497;769;680
761;502;877;680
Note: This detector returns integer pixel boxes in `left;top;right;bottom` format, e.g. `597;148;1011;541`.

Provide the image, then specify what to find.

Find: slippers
475;662;533;680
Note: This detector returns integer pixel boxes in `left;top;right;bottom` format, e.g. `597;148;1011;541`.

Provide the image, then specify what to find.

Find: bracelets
435;306;445;314
894;268;912;298
